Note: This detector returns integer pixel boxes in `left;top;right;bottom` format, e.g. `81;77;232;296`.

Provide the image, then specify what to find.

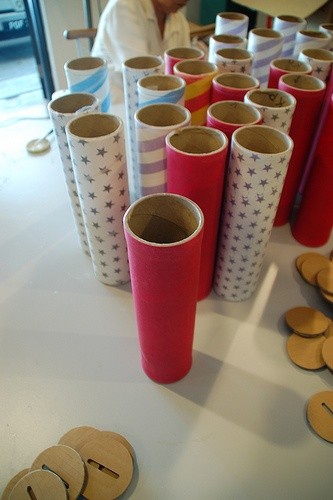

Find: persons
90;0;190;104
